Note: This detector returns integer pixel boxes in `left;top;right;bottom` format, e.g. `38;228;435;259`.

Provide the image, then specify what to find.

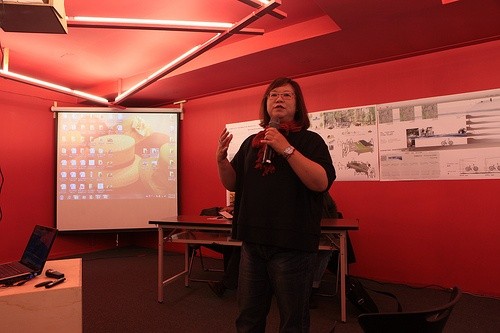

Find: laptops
0;224;59;281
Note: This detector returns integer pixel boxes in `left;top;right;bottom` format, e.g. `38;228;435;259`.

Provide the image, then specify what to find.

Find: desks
0;258;83;333
149;214;359;323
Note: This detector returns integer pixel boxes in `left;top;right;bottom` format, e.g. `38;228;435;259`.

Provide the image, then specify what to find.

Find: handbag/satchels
345;275;379;315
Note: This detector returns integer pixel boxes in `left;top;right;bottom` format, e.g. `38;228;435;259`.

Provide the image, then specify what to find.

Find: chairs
358;286;462;333
189;207;226;284
319;212;356;296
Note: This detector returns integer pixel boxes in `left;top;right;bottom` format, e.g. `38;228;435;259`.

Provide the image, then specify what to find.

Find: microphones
262;116;281;166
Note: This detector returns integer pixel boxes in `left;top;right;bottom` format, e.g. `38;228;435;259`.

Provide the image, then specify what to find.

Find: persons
215;77;336;333
208;190;337;308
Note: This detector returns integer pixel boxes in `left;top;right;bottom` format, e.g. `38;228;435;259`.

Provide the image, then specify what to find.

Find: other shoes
309;297;316;308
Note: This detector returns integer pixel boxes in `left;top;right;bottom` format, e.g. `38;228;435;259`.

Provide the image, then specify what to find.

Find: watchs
283;145;296;160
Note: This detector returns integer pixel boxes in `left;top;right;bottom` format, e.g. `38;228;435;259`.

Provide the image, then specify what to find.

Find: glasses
266;90;296;100
